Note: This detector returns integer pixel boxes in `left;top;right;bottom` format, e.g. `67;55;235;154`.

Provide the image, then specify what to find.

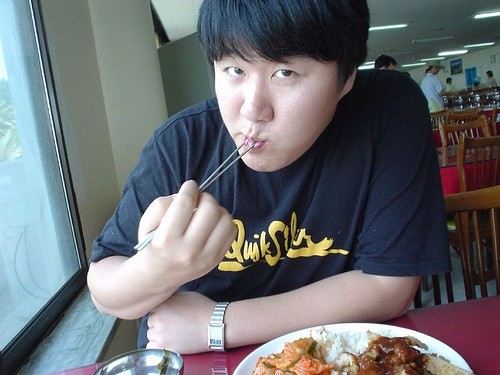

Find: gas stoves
453;101;500;111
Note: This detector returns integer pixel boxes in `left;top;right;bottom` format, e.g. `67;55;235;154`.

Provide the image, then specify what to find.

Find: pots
442;91;500;106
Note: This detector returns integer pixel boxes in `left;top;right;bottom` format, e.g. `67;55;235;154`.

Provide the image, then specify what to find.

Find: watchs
207;301;229;352
210;356;228;375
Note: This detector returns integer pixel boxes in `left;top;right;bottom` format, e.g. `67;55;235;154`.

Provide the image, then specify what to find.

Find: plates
232;323;473;375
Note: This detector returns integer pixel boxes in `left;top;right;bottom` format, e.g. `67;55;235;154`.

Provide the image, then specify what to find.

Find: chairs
413;87;500;312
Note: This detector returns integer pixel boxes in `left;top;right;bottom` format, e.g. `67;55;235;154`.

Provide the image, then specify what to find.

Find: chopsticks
132;141;255;251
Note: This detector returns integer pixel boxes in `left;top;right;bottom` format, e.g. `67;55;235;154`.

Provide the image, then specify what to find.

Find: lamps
412;35;456;43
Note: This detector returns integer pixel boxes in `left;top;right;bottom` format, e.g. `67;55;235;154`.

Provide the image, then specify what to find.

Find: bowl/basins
92;349;185;375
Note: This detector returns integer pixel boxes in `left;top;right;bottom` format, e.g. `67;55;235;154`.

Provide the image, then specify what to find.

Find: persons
375;54;397;71
87;0;443;355
444;77;455;94
486;70;497;87
420;60;446;114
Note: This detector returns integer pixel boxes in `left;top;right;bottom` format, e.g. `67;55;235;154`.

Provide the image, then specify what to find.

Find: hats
428;60;442;68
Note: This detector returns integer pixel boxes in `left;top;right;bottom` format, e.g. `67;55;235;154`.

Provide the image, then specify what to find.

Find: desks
431;112;500;146
437;144;500;194
53;297;500;375
